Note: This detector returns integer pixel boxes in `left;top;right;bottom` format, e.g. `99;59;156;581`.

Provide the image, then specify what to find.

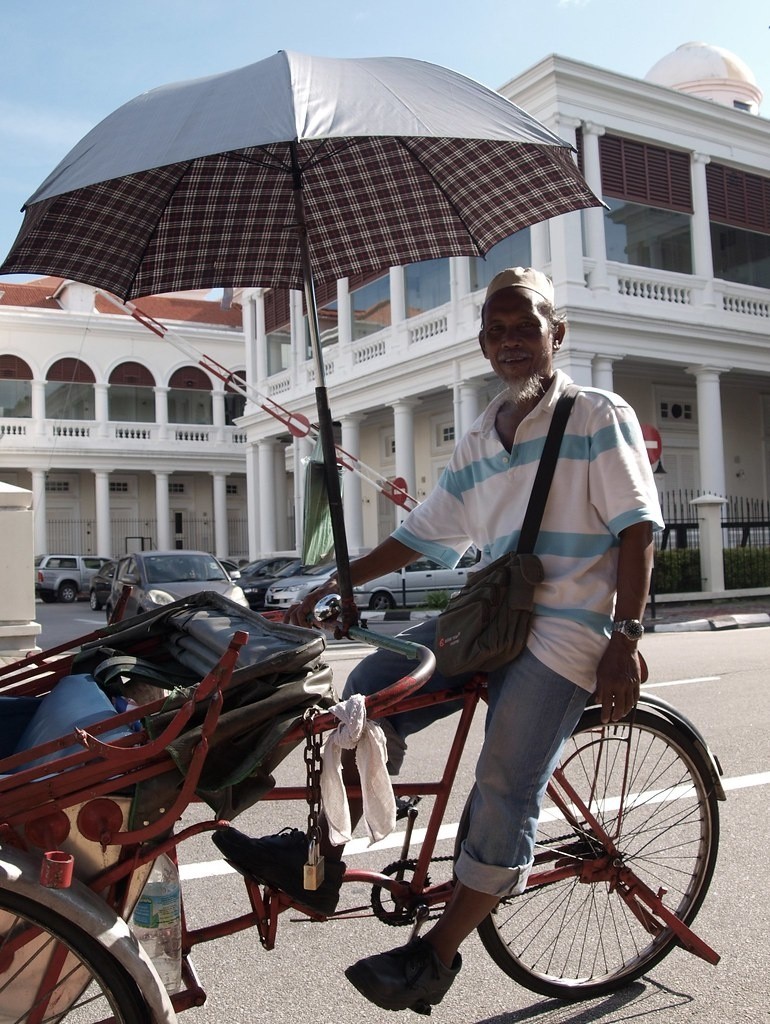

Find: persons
210;264;667;1018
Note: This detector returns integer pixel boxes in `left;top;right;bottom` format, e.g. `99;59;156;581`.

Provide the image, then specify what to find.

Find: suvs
34;553;114;604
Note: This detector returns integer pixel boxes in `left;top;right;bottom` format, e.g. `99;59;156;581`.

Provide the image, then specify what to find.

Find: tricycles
0;586;734;1024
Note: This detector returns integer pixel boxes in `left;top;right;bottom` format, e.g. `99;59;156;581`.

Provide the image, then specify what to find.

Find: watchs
614;619;644;641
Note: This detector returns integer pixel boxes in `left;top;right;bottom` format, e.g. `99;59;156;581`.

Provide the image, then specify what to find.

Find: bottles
131;854;182;997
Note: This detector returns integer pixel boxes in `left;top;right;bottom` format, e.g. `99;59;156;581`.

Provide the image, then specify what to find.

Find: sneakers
345;936;462;1011
212;824;346;916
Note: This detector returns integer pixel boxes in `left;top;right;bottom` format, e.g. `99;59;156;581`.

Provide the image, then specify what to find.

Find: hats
484;267;554;310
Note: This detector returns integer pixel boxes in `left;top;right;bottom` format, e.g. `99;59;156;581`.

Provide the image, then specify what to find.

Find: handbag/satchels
432;550;544;677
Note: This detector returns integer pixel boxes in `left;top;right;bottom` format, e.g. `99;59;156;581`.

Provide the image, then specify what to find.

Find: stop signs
392;478;409;505
287;414;312;439
641;424;663;467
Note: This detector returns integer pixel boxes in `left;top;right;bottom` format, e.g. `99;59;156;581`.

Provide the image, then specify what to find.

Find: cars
266;553;479;613
106;552;251;625
90;561;123;611
240;558;325;609
230;556;301;605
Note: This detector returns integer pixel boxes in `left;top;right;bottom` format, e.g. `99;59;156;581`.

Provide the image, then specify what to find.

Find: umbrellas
0;50;612;619
